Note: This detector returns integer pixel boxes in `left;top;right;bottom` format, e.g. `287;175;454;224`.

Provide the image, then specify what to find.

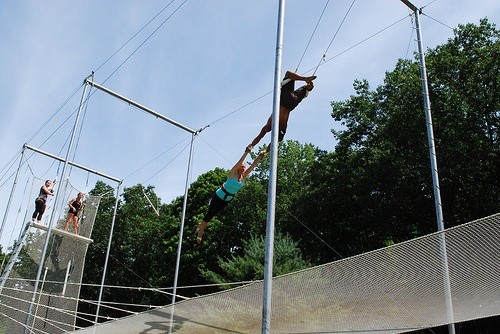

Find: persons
245;70;317;156
32;179;55;222
63;192;84;235
194;143;265;244
